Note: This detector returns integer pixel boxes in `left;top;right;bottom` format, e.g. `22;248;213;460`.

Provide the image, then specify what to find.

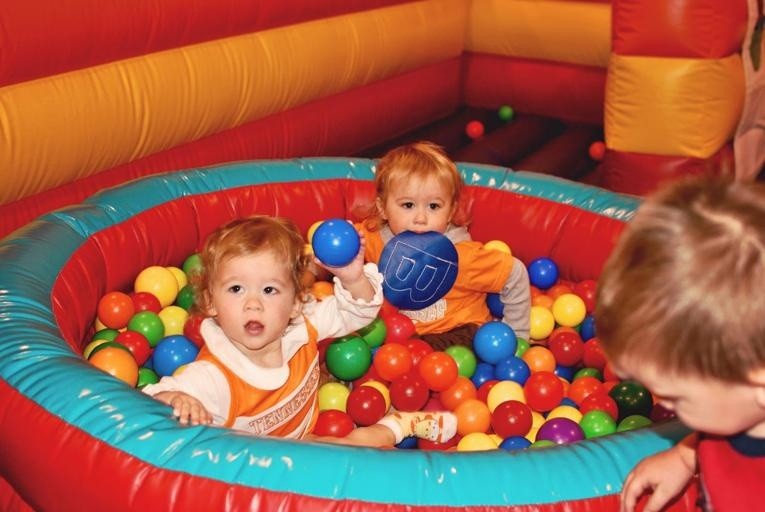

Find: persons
141;215;458;450
593;177;765;512
300;139;531;353
